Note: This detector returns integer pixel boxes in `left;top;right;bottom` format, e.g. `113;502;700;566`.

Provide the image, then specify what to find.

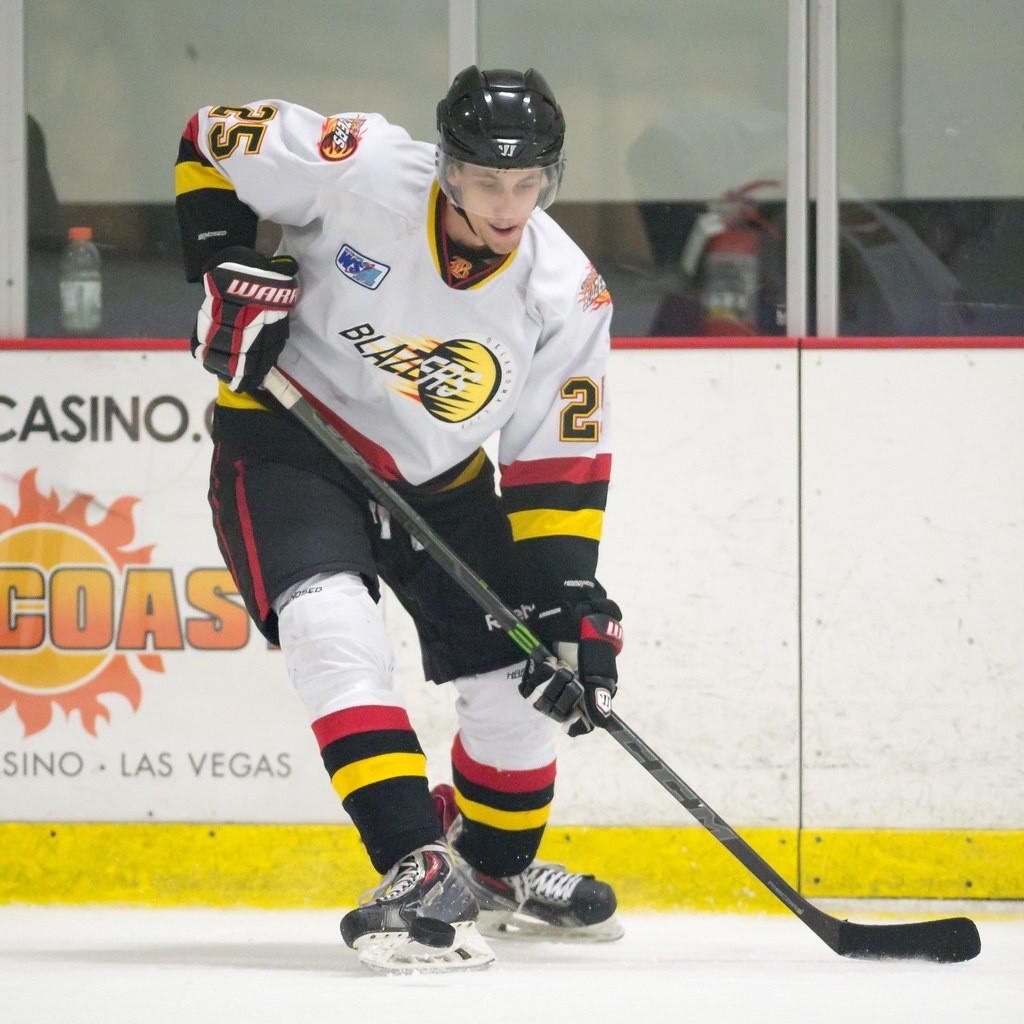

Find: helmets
434;62;567;168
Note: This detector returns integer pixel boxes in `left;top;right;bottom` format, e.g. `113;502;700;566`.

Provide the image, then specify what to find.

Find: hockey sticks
261;364;984;966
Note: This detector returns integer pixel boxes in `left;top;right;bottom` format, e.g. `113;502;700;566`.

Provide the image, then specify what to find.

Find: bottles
60;228;102;334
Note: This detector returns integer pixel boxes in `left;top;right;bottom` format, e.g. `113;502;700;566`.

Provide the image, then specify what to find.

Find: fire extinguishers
682;179;784;338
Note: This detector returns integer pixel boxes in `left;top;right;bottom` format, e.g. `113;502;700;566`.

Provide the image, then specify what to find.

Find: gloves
517;573;624;739
190;245;299;394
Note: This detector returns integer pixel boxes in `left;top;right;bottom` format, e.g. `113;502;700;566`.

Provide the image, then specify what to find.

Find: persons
175;65;622;979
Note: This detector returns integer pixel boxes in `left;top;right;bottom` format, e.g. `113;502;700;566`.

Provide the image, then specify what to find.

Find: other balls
412;915;457;951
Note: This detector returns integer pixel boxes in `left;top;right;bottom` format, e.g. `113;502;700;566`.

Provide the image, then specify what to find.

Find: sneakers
443;830;627;943
339;840;495;972
